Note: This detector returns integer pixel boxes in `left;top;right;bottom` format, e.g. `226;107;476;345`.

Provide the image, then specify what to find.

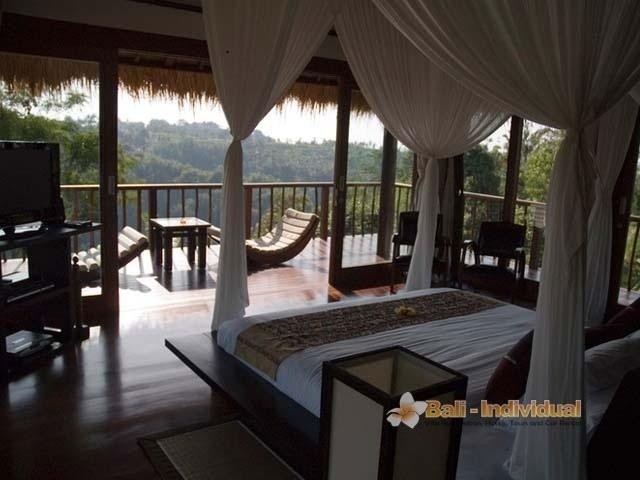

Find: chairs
71;224;149;280
165;287;640;480
458;221;527;289
389;211;452;293
208;207;321;266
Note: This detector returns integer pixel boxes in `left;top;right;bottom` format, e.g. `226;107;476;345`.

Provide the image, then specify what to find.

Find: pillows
478;326;623;428
606;297;640;336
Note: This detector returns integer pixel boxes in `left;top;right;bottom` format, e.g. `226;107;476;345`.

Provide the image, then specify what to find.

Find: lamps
318;345;469;480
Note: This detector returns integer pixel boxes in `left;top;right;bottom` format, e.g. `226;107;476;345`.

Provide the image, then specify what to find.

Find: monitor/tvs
0;139;61;240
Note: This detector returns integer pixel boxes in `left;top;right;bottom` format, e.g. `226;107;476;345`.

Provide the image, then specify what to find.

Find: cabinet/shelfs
1;220;104;386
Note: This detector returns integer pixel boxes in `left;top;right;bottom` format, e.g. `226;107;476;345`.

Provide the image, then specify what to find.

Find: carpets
136;413;315;480
